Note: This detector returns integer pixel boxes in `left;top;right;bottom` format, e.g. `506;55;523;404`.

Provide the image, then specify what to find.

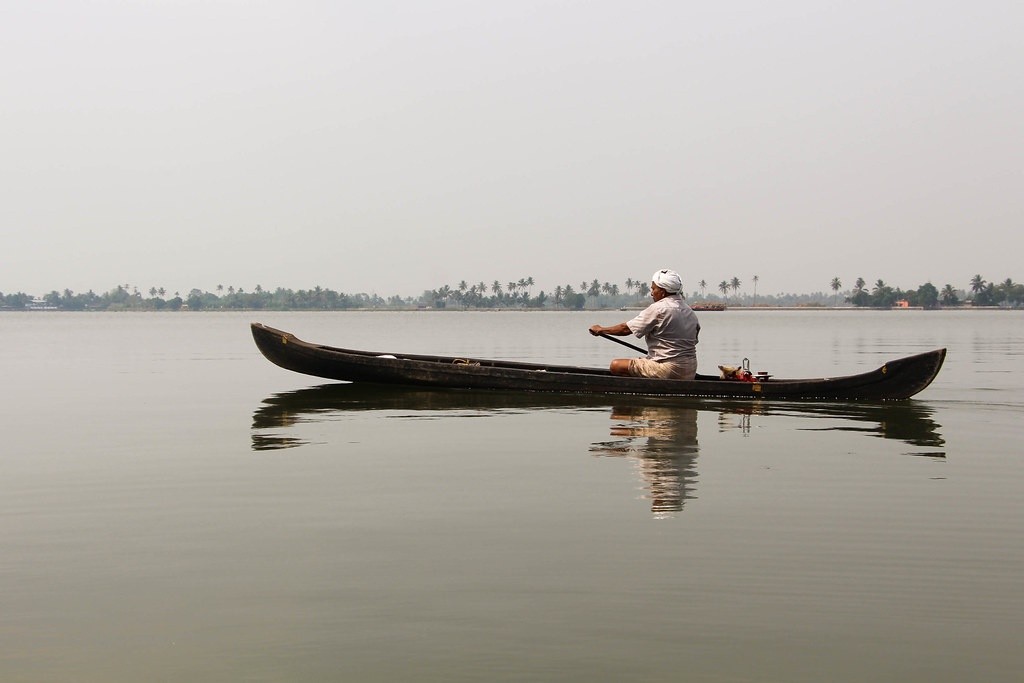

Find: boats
250;320;946;404
690;304;725;311
250;381;946;462
620;307;627;311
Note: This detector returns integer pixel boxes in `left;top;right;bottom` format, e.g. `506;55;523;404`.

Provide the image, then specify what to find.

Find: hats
652;269;682;293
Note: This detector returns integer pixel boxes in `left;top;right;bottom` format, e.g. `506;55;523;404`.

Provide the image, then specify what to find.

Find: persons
589;269;700;379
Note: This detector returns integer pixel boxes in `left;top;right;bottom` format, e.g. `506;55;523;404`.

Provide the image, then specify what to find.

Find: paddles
588;328;649;355
